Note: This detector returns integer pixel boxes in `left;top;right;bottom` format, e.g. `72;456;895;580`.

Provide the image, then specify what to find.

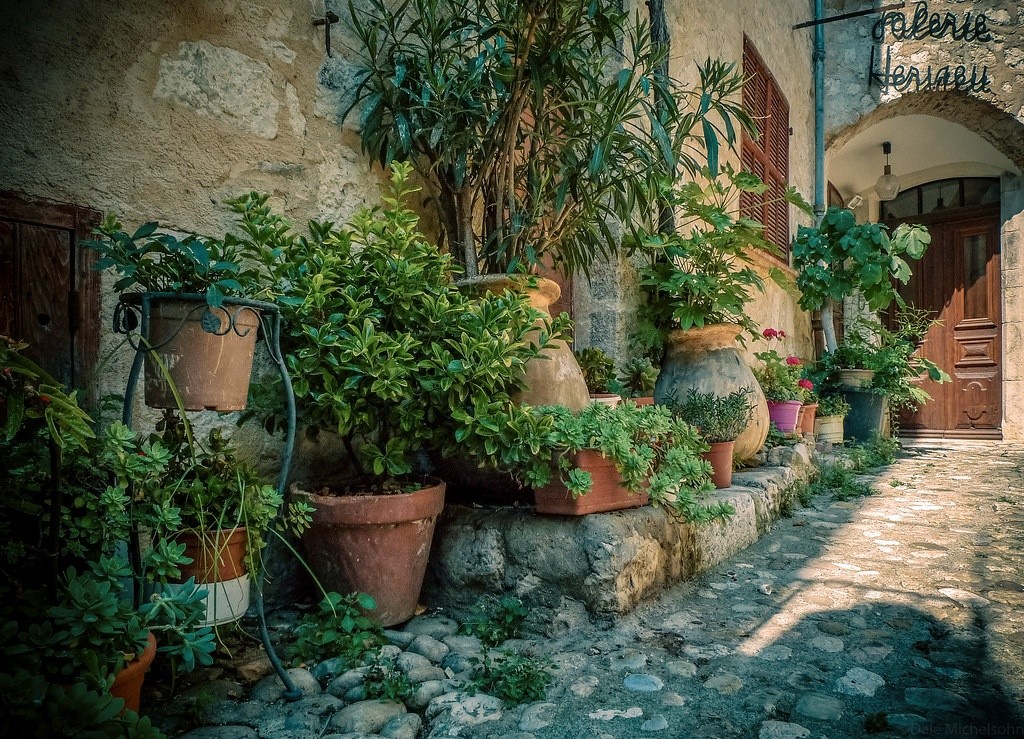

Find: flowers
748;328;813;402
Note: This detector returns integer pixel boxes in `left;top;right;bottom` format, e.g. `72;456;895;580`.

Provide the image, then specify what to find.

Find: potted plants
0;1;954;739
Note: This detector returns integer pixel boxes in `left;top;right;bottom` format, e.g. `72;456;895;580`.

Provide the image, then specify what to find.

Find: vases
767;400;802;433
